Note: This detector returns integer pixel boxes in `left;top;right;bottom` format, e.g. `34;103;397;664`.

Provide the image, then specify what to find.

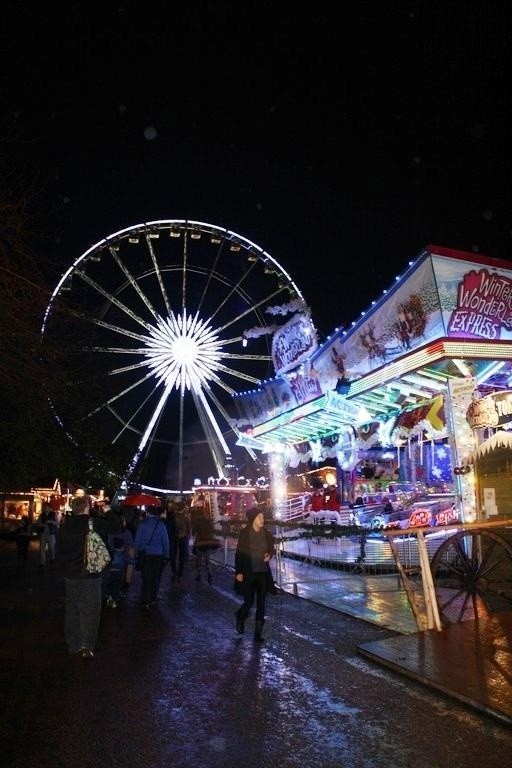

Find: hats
246;508;262;523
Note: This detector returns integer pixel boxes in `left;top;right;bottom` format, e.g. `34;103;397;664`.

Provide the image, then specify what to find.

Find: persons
15;496;219;660
234;508;276;641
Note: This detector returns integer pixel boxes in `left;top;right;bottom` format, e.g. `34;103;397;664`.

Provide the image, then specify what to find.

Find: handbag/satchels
134;554;147;571
84;530;112;574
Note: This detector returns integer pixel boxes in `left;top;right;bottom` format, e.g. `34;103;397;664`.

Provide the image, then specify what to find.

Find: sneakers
170;574;183;583
78;645;93;659
195;574;214;584
144;603;152;610
105;596;117;608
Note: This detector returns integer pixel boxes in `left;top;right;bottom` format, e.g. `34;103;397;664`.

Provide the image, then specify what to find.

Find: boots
236;605;248;634
254;611;265;642
